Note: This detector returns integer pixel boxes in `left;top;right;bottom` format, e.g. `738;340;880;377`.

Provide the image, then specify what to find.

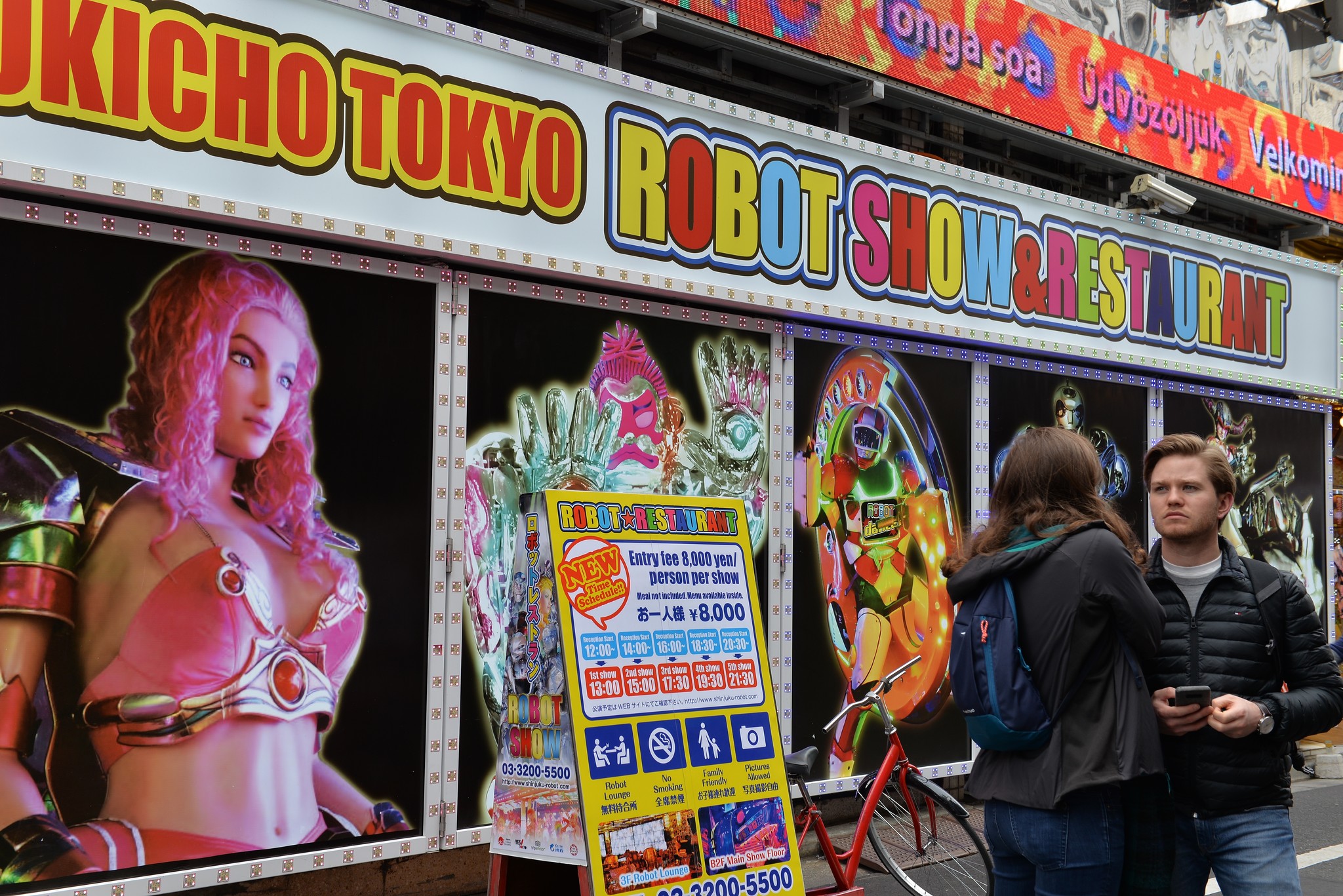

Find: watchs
1253;700;1275;735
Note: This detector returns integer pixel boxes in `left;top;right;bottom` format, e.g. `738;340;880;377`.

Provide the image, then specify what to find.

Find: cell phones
1174;686;1211;710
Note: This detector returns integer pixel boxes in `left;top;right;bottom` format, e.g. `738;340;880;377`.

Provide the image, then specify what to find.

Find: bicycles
784;654;995;896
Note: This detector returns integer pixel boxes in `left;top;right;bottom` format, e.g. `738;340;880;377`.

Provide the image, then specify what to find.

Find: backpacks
949;577;1115;756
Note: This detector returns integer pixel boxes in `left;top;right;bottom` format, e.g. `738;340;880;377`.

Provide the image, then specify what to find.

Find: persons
941;426;1168;896
1137;434;1342;896
1;252;411;883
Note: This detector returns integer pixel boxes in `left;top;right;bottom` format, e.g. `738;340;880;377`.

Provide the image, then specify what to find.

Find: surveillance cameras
1130;174;1197;216
